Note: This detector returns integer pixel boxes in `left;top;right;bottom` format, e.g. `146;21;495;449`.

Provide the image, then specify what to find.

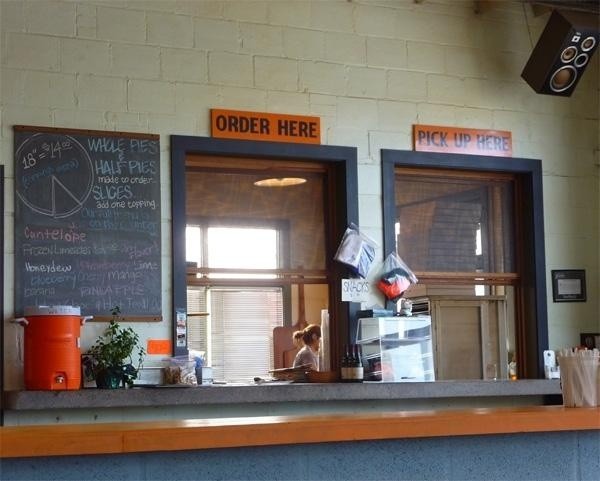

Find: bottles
341;343;364;383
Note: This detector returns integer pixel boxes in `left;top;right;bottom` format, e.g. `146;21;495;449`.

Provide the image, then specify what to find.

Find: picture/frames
551;269;587;303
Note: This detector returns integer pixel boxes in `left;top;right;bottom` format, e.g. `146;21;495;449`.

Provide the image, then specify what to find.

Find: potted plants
81;306;148;389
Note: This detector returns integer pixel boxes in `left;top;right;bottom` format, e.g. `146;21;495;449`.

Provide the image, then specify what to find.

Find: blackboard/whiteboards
13;125;163;323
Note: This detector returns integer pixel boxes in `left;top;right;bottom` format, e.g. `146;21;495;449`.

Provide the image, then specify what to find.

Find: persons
292;324;321;370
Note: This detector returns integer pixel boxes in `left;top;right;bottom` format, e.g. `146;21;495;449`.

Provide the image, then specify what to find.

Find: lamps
254;172;308;188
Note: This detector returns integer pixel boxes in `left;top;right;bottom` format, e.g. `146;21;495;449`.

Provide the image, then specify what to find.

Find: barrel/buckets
11;302;94;391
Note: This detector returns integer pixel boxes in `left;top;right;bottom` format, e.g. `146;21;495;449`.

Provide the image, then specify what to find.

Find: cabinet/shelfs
355;315;436;383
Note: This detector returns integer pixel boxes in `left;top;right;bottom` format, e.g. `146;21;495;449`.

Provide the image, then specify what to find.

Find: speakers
520;8;600;97
